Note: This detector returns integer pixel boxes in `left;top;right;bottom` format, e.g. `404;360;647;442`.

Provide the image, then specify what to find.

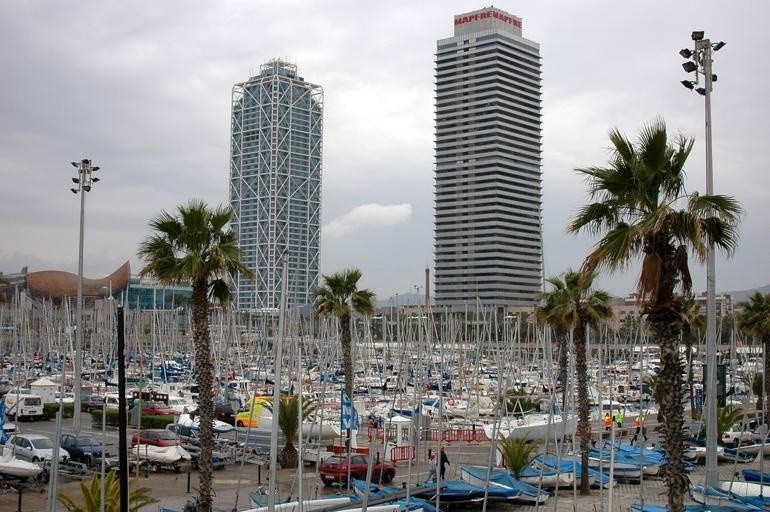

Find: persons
440;445;451;480
604;412;611;432
634;411;650;442
423;447;437;484
615;408;623;428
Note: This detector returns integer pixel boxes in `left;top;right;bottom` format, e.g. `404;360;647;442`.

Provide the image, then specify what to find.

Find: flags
341;388;359;431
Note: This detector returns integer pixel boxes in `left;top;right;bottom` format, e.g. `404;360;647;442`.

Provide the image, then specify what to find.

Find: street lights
679;28;728;494
69;156;102;435
